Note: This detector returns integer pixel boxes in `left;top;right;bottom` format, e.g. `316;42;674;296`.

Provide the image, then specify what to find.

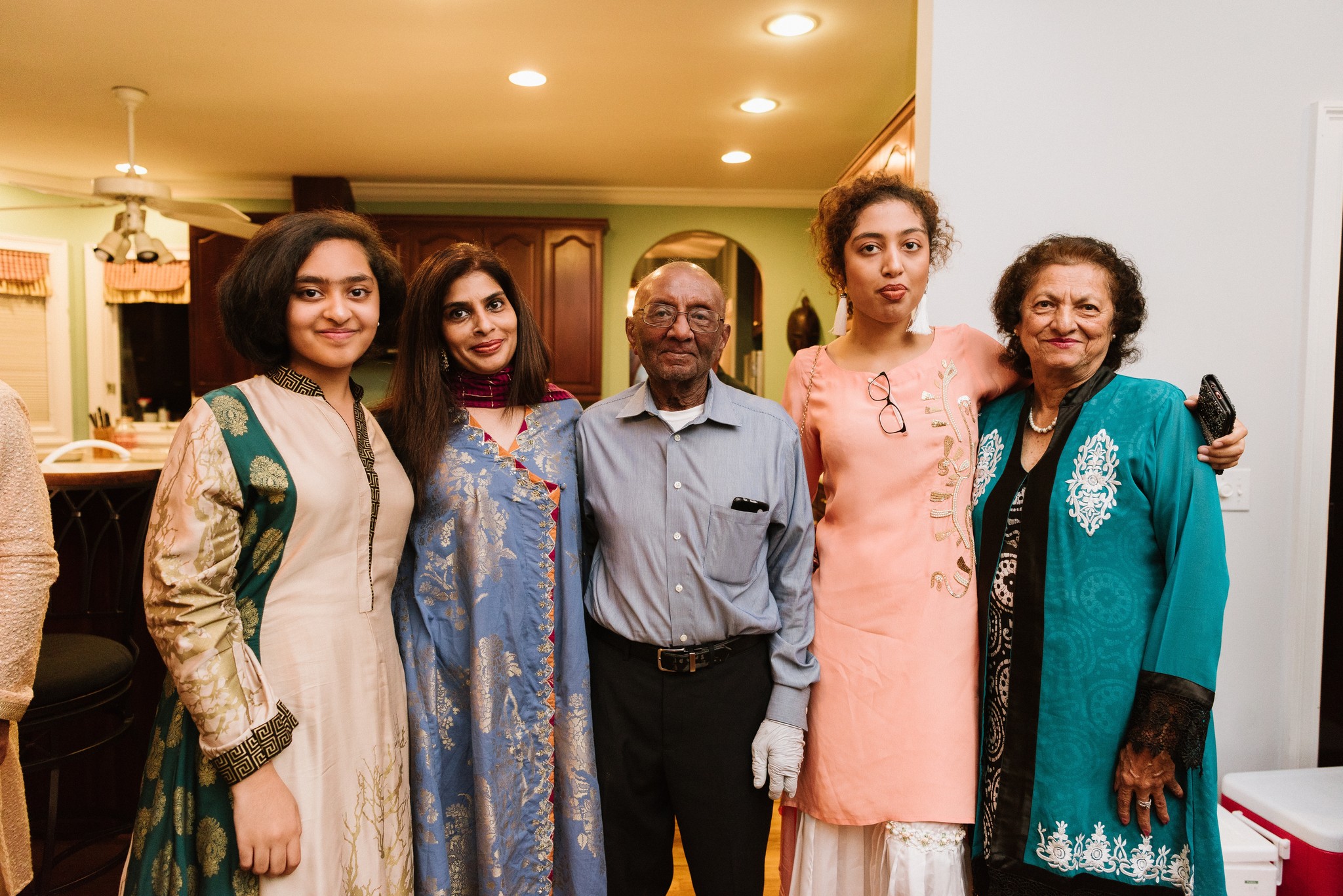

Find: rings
1136;798;1151;809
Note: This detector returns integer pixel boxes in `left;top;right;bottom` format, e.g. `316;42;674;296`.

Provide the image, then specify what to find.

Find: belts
591;625;762;674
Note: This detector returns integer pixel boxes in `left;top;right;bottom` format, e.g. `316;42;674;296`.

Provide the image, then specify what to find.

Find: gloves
752;718;805;801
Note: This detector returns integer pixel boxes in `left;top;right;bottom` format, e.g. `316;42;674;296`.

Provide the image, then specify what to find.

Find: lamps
92;199;174;269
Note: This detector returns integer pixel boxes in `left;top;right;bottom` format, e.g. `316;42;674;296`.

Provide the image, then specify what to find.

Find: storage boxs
1222;766;1343;896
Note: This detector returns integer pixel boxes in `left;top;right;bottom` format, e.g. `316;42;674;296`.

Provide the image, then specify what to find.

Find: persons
575;261;821;896
970;233;1230;896
365;240;607;896
779;169;1248;896
711;349;757;396
0;381;60;896
118;207;416;896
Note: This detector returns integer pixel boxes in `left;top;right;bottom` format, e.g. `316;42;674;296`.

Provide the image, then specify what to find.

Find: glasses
870;371;908;435
635;303;725;333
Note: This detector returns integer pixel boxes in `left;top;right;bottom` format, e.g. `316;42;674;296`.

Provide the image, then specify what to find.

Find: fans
0;86;263;240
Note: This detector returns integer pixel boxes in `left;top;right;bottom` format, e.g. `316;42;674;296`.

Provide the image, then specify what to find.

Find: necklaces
1028;405;1058;434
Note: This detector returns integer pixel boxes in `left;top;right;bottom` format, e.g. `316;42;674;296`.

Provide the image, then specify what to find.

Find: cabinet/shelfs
189;212;610;400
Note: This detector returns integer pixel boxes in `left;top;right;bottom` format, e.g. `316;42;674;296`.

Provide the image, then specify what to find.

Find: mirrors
626;229;765;398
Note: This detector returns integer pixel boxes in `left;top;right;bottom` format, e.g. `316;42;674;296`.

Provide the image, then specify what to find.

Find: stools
18;632;146;896
